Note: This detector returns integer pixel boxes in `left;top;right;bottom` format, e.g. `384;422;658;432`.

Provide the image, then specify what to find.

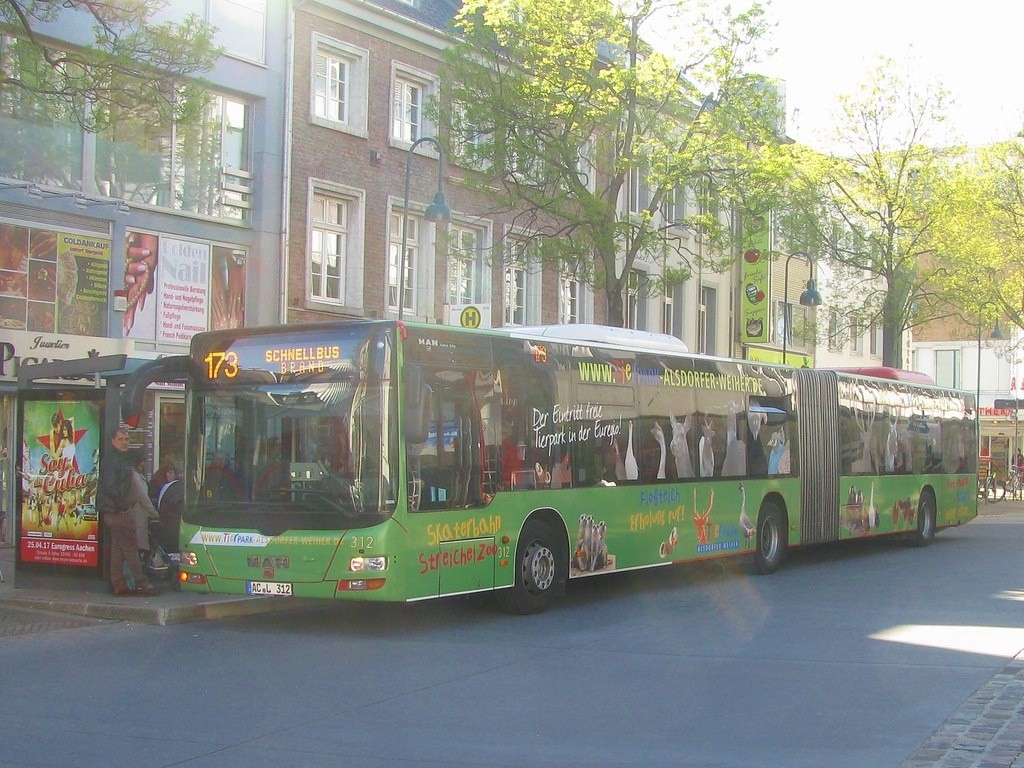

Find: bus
120;313;986;618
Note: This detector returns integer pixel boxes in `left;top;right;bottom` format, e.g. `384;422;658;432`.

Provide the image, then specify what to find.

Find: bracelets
233;249;247;255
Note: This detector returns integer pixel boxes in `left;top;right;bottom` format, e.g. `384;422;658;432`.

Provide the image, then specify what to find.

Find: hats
131;452;146;465
159;464;175;471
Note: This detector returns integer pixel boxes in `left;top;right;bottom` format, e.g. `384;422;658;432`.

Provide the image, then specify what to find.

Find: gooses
625;420;638;480
651;422;667;480
869;481;876;529
739;484;757;549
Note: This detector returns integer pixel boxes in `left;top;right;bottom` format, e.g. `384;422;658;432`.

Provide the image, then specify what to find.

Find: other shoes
136;587;161;596
112;589;137;597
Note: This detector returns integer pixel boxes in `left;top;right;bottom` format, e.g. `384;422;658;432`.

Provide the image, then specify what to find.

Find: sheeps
699;419;716;477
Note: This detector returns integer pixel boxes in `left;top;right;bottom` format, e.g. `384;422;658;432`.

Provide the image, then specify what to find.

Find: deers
850;399;877;473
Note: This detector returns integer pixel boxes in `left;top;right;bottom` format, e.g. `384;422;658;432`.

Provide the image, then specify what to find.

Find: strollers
139;515;183;594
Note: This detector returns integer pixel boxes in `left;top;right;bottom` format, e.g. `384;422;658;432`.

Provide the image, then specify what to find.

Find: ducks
610;438;627;480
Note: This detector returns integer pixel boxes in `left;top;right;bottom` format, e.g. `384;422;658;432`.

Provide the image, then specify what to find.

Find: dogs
668;411;696;479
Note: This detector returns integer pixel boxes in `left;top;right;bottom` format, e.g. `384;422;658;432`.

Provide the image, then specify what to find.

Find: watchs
233;255;246;266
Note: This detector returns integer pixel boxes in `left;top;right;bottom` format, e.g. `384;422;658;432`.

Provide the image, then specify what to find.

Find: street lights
783;252;823;370
398;136;454;322
977;301;1005;459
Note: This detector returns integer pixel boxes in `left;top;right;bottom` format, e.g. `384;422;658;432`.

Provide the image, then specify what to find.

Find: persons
124;231;158;337
500;438;523;489
214;247;245;330
209;449;229;470
100;427;161;596
981;445;989;456
148;454;184;570
127;448;159;592
1012;449;1024;482
267;435;283;466
31;231;57;259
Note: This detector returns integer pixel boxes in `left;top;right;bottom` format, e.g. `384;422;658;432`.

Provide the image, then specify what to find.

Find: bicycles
976;461;1006;504
1005;464;1024;501
